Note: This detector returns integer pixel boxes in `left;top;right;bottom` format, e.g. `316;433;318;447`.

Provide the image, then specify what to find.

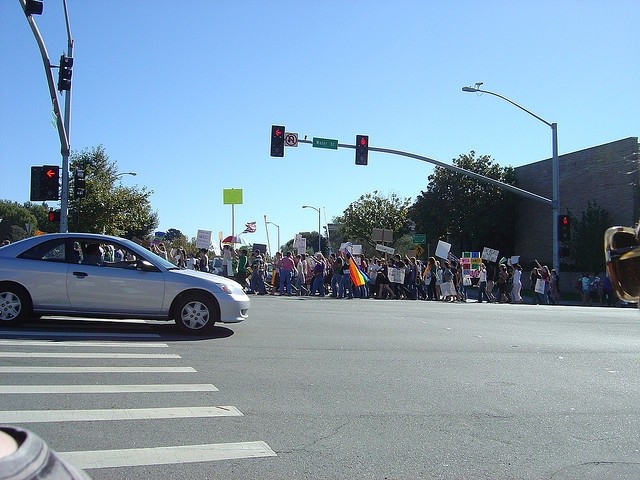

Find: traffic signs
311;135;342;152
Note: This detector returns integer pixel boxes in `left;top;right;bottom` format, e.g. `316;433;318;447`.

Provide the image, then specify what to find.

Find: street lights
302;206;321;251
107;173;137;179
462;82;560;276
267;222;279;252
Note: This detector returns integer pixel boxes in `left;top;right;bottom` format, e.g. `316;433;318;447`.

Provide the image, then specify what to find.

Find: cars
1;232;250;336
265;262;274;283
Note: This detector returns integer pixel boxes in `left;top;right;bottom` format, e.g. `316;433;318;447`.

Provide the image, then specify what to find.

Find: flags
345;248;371;287
242;221;256;233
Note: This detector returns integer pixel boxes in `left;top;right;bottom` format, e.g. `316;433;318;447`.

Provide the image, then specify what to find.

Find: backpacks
575;281;582;292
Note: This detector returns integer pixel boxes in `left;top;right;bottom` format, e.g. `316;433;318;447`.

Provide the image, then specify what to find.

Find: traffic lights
43;165;59;201
355;135;368;165
558;215;570;241
58;55;73;91
270;125;285;157
74;169;85;198
25;0;44;18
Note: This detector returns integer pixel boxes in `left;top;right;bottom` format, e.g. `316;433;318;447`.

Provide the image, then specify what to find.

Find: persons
495;262;508;304
423;257;437;301
233;249;247;293
510;262;524;304
219;238;231;277
323;254;337;295
311;252;325;296
435;261;441;301
73;242;83;264
250;249;265;295
300;254;307;296
578;272;616;307
442;262;457;302
278;252;295;296
199;248;209;272
330;251;344;299
270;252;282;295
393;255;407;300
295;255;305;297
378;258;395;298
156;243;167;261
151;245;156;253
476;263;493;303
535;260;551;304
547;269;560;304
115;247;124;261
360;254;368;298
404;253;418;300
530;268;542;305
337;253;353;299
403;258;409;299
260;253;264;259
450;261;457;301
2;240;13;246
103;247;111;262
